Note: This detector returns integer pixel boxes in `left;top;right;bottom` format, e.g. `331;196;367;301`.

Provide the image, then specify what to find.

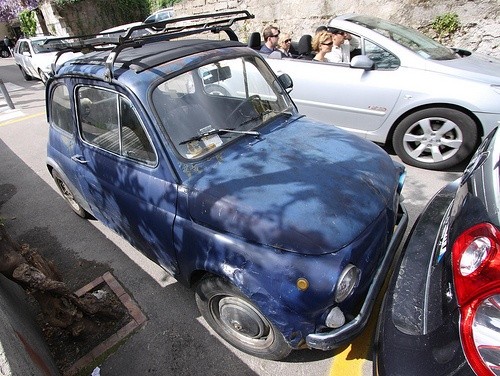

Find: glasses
268;30;280;38
283;39;291;44
337;32;344;36
321;41;333;46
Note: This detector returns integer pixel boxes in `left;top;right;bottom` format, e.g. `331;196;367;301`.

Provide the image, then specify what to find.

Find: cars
93;21;153;49
43;9;410;360
0;38;16;58
373;122;500;376
13;35;84;86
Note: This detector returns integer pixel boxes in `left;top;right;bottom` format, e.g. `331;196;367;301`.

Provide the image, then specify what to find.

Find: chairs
91;125;151;160
166;102;219;148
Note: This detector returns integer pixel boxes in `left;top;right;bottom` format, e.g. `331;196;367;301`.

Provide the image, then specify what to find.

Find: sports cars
185;12;500;171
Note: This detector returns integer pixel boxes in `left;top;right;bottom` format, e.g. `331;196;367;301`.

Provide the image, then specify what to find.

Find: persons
4;36;15;58
311;26;359;63
260;26;292;58
